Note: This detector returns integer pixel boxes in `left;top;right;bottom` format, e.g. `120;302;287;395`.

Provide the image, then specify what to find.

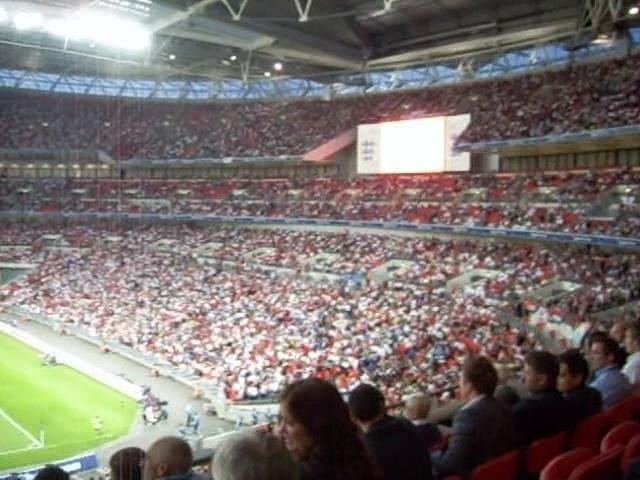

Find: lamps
591;33;613;44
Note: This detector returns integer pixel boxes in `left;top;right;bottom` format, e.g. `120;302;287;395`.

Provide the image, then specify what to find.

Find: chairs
0;54;640;480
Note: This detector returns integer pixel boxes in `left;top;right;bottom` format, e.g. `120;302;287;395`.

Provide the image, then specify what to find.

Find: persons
1;58;640;479
